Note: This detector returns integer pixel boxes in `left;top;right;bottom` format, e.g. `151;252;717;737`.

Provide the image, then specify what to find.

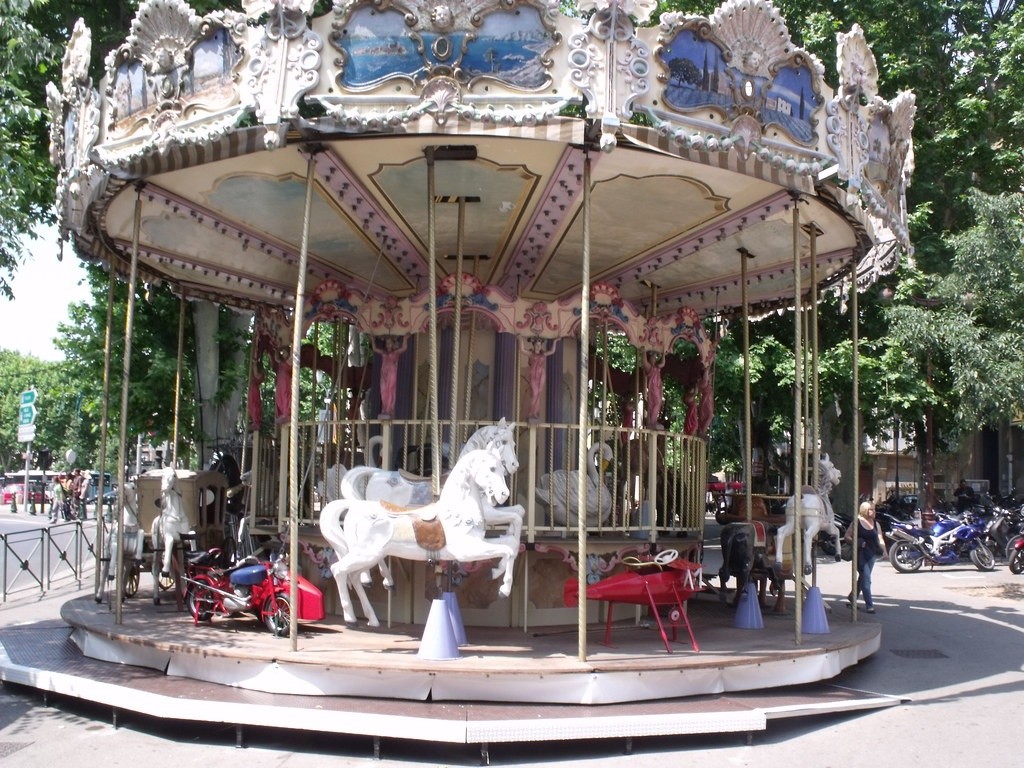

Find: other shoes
866;608;876;614
846;603;860;609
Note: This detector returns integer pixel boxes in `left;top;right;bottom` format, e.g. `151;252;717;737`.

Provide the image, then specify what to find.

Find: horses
94;466;197;602
315;416;526;623
774;453;843;573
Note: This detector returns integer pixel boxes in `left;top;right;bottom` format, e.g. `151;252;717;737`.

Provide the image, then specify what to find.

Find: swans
535;441;614;528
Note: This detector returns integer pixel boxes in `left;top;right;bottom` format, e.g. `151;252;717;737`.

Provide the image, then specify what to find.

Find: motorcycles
816;486;1024;575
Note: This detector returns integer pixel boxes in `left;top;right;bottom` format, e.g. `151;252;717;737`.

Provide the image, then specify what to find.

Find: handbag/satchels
841;542;853;561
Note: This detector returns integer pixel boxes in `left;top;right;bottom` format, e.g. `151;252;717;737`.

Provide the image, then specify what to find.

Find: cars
2;483;49;504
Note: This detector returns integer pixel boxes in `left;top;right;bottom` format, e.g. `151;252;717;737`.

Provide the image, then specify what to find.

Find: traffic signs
17;389;39;442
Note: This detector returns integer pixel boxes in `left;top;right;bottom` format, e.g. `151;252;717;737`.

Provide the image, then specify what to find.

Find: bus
0;468;113;504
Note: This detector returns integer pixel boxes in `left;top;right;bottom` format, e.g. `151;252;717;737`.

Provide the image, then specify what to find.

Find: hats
80;469;93;479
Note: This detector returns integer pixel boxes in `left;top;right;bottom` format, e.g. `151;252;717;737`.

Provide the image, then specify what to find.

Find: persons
48;469;93;524
844;500;888;613
953;479;974;512
246;333;715;440
